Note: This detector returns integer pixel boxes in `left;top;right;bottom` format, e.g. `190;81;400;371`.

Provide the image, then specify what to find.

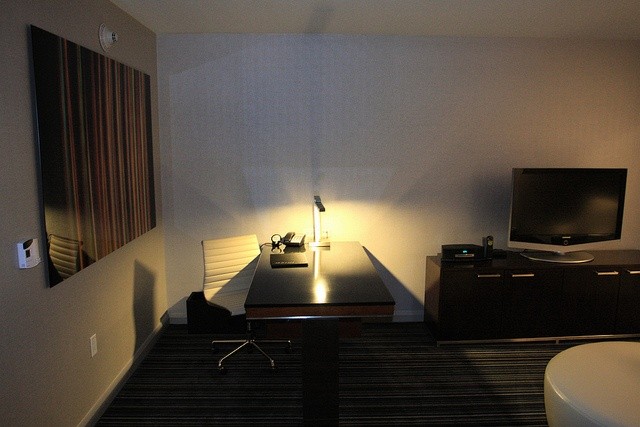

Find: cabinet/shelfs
424;249;640;347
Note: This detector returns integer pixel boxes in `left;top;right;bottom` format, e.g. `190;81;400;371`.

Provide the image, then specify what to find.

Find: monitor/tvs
507;167;628;263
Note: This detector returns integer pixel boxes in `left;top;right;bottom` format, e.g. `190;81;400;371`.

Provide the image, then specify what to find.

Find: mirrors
23;21;157;289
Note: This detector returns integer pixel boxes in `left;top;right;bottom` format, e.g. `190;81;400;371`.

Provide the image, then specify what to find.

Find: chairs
47;234;84;280
201;234;293;372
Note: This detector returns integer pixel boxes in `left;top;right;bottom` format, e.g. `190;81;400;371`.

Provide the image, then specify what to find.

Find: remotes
486;235;494;260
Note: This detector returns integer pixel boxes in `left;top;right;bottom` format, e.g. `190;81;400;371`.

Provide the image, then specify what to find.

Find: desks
242;239;396;425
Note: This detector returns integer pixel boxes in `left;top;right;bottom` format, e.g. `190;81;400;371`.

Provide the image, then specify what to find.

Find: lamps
309;196;331;247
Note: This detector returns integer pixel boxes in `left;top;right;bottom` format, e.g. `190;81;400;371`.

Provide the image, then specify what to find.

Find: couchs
543;341;640;427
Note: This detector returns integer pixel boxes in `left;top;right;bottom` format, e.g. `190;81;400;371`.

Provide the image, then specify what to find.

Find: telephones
282;232;306;247
